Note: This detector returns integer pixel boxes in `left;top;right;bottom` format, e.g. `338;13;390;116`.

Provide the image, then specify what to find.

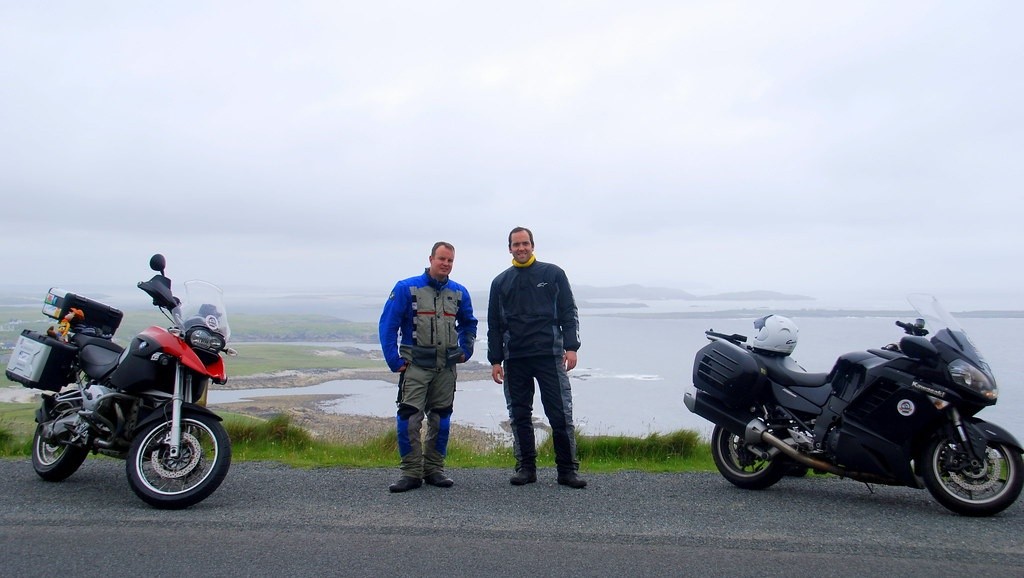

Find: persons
379;241;479;492
485;227;588;487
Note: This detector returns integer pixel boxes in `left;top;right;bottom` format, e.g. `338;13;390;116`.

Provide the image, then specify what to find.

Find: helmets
753;315;799;356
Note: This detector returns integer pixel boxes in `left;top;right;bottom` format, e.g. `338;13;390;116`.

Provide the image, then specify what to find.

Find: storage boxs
6;328;79;393
41;287;124;339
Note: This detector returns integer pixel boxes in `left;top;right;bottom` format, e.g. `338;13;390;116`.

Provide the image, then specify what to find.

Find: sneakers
558;473;587;487
424;472;454;487
510;469;537;485
389;476;422;492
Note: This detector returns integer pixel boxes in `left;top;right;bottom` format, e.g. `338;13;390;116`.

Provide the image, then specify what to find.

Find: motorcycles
4;253;239;512
682;292;1023;519
